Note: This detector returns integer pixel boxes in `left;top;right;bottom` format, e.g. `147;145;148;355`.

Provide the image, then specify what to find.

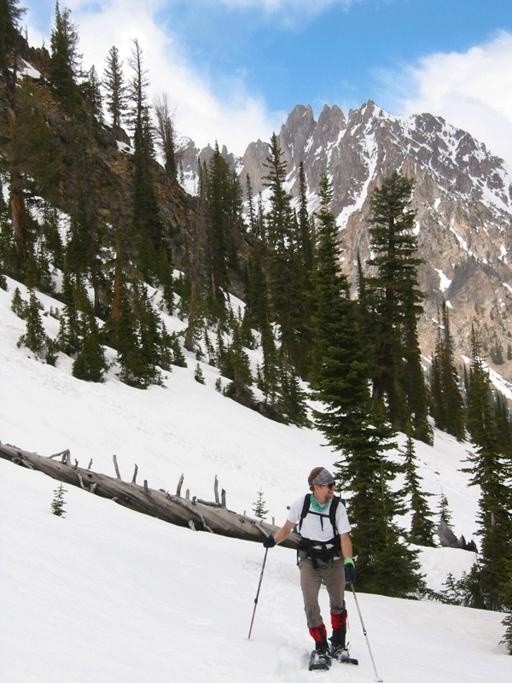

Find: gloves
344;557;356;584
264;534;276;547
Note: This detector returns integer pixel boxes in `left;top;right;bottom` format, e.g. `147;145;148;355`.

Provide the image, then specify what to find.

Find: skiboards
309;646;358;670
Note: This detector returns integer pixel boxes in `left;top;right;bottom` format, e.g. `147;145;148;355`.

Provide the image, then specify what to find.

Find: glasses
322;482;335;490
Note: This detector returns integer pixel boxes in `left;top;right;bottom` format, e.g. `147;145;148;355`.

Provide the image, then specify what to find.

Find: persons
262;466;355;662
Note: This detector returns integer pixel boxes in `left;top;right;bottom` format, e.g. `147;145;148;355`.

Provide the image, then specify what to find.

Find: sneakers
331;644;349;661
312;647;330;665
